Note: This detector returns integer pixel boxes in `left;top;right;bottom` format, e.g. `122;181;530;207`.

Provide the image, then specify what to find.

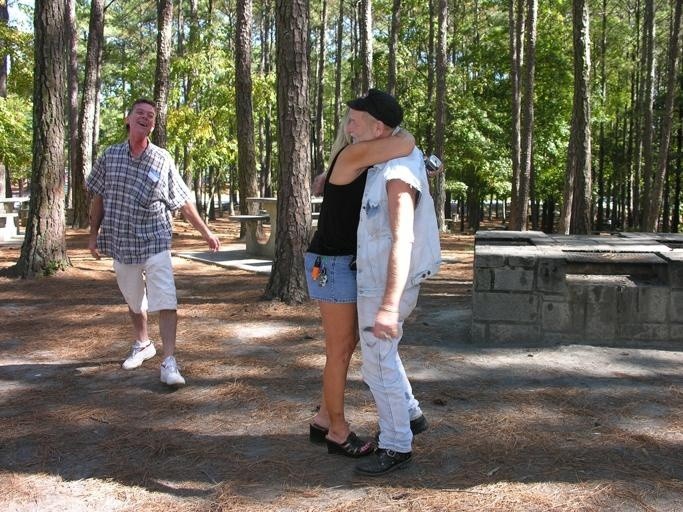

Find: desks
244;196;323;251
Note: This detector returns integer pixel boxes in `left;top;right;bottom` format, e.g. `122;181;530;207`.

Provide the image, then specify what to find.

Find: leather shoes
354;446;413;476
372;413;429;441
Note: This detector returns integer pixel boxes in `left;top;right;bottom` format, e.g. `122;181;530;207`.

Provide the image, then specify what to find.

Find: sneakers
119;338;158;373
158;355;187;388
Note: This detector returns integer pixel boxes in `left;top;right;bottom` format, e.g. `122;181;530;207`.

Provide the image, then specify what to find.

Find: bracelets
376;304;400;316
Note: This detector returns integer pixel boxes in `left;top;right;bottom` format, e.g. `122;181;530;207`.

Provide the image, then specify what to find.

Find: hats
344;88;404;129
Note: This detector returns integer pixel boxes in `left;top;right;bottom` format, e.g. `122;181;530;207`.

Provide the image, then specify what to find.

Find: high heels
324;430;376;458
309;421;330;445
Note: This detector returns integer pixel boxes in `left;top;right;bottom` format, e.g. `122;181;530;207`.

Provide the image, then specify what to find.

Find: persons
84;99;221;388
300;106;445;462
310;86;443;477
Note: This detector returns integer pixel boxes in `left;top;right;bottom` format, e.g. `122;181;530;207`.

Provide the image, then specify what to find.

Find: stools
226;212;319;257
